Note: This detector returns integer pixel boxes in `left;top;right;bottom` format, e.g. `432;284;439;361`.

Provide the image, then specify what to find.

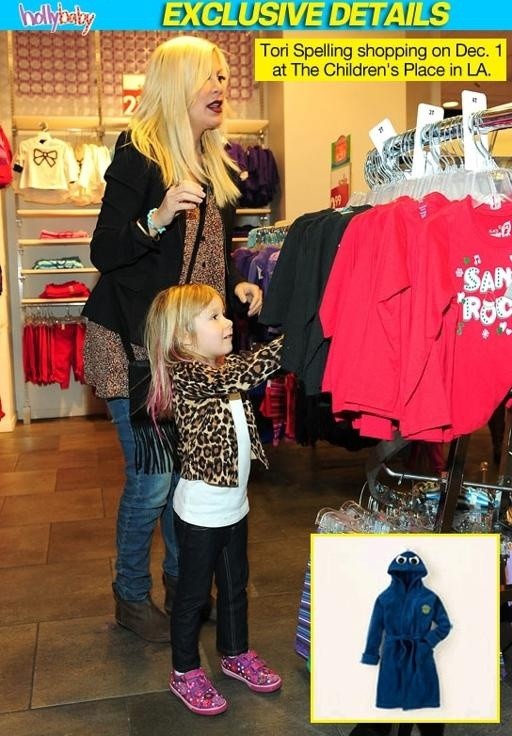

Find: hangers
23;121;109;151
238;227;292;260
21;305;89;330
298;109;512;229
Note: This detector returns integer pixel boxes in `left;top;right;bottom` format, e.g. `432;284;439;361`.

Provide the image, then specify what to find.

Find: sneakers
220;650;283;693
168;666;228;716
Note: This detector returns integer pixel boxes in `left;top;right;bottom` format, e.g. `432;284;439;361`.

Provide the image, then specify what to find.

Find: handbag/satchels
128;360;182;426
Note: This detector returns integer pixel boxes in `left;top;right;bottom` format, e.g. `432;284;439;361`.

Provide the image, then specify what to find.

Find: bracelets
146;206;167;235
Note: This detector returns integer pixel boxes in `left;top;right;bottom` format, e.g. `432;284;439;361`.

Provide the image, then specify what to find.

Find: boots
112;583;171;642
162;572;220;624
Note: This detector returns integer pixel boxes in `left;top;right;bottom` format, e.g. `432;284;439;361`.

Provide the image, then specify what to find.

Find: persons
79;36;264;642
145;283;284;718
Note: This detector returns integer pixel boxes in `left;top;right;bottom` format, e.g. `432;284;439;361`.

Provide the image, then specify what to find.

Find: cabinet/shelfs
10;114;272;424
